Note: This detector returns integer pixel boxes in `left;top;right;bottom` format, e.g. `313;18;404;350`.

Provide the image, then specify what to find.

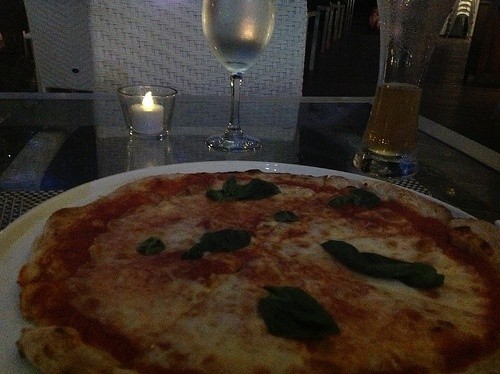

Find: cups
352;0;462;180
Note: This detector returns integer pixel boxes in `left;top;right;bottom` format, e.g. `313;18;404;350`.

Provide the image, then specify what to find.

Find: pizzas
16;170;499;373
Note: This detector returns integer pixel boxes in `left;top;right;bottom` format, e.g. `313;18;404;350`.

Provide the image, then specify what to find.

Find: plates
0;160;481;374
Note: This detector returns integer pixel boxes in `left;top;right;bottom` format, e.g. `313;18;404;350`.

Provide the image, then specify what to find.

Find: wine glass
202;0;276;152
